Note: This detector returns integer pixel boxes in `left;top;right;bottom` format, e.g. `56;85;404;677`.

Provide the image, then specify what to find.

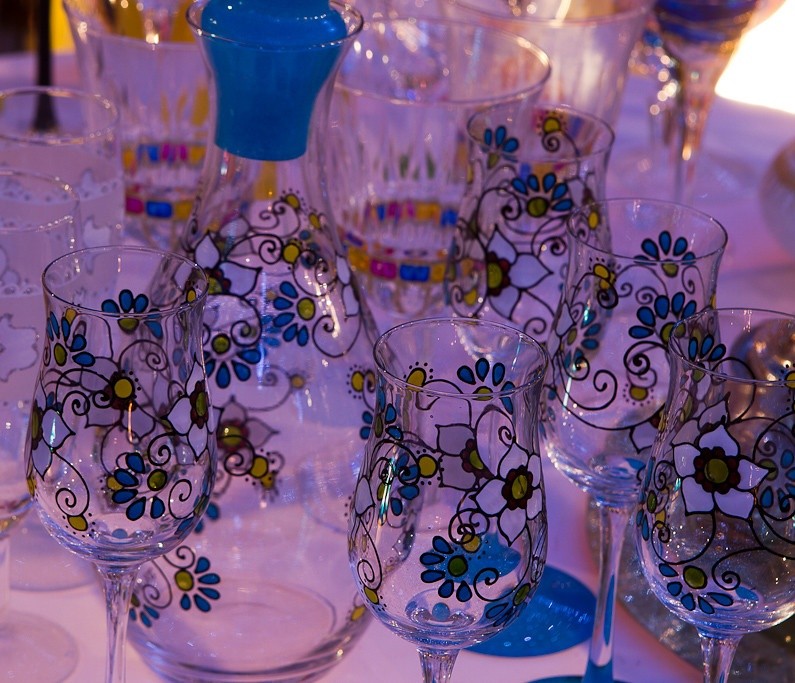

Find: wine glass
24;244;217;682
637;307;794;682
530;196;729;683
346;317;550;683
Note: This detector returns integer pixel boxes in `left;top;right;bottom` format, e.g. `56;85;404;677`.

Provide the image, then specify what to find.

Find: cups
63;0;777;531
2;84;124;594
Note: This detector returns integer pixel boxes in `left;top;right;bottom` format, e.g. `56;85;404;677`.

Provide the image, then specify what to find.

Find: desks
0;51;795;683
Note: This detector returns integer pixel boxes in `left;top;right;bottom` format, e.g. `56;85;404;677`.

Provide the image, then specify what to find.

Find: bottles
106;0;418;683
